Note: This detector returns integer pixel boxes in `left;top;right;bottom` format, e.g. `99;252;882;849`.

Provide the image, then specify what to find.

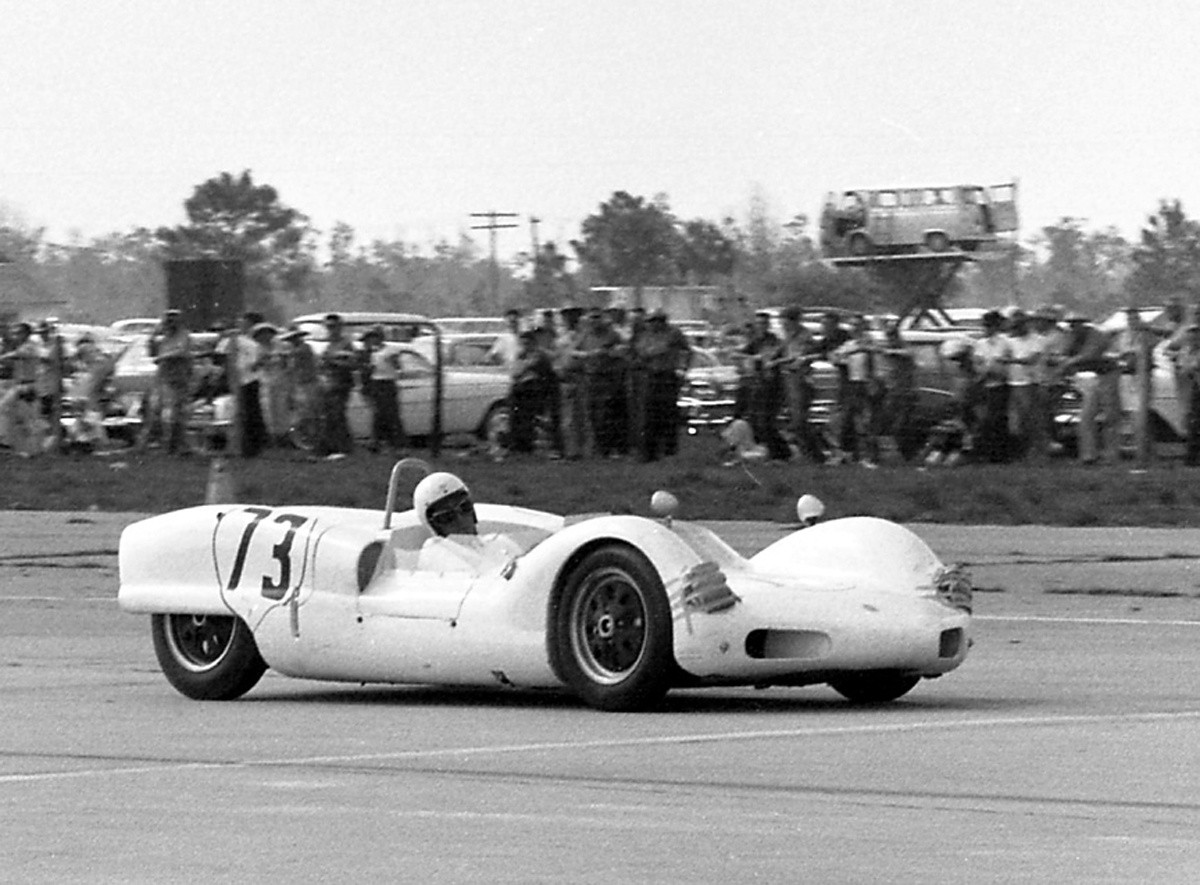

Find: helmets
413;471;479;538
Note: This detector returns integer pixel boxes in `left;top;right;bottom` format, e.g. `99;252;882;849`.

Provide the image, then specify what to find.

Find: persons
0;308;408;462
483;304;693;464
412;471;521;572
723;305;1200;474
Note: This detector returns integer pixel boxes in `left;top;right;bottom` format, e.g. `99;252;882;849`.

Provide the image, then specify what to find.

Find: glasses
430;495;472;525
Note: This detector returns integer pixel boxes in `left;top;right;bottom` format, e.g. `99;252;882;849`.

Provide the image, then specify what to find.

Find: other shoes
861;458;878;469
546;451;563;461
565;453;582;465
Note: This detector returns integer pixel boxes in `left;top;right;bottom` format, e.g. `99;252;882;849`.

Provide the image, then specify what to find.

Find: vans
834;183;1020;255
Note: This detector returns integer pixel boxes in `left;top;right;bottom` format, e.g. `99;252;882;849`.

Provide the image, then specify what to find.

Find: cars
22;305;1200;457
117;458;976;711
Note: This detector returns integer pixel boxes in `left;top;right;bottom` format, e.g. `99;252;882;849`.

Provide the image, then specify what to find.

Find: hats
981;306;1094;329
246;322;278;340
35;323;56;333
278;324;308;341
